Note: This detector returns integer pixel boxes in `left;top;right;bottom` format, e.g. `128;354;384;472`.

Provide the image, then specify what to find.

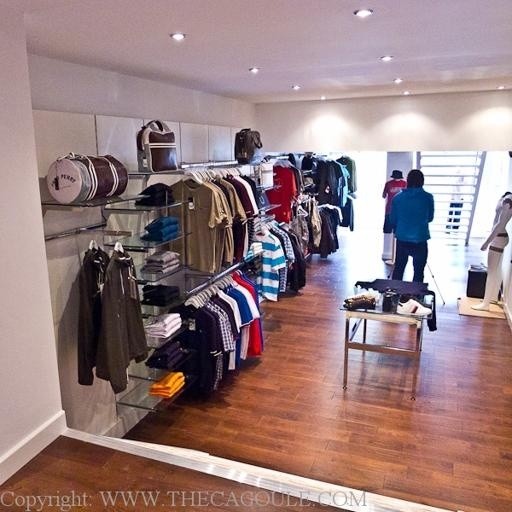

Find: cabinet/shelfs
104;171;199;412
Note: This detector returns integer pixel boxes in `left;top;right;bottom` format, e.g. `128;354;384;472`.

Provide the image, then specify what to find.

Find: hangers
184;266;243;309
87;230;124;254
253;218;279;235
272;155;295;168
182;158;242;185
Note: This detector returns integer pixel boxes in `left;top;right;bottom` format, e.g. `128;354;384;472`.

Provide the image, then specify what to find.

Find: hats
390;170;404;178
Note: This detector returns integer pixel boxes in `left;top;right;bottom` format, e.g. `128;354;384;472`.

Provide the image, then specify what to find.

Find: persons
389;170;435;304
471;192;512;311
383;170;407;233
444;174;466;234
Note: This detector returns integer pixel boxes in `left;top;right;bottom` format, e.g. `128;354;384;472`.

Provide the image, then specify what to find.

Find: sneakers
396;298;433;316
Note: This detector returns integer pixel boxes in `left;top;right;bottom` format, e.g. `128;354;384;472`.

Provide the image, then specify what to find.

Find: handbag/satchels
382;287;400;313
343;293;376;310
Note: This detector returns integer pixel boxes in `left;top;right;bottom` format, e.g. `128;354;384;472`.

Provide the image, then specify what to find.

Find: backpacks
136;119;179;174
234;127;263;164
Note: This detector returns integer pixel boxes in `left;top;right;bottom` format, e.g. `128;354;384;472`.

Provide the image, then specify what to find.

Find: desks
339;289;433;400
